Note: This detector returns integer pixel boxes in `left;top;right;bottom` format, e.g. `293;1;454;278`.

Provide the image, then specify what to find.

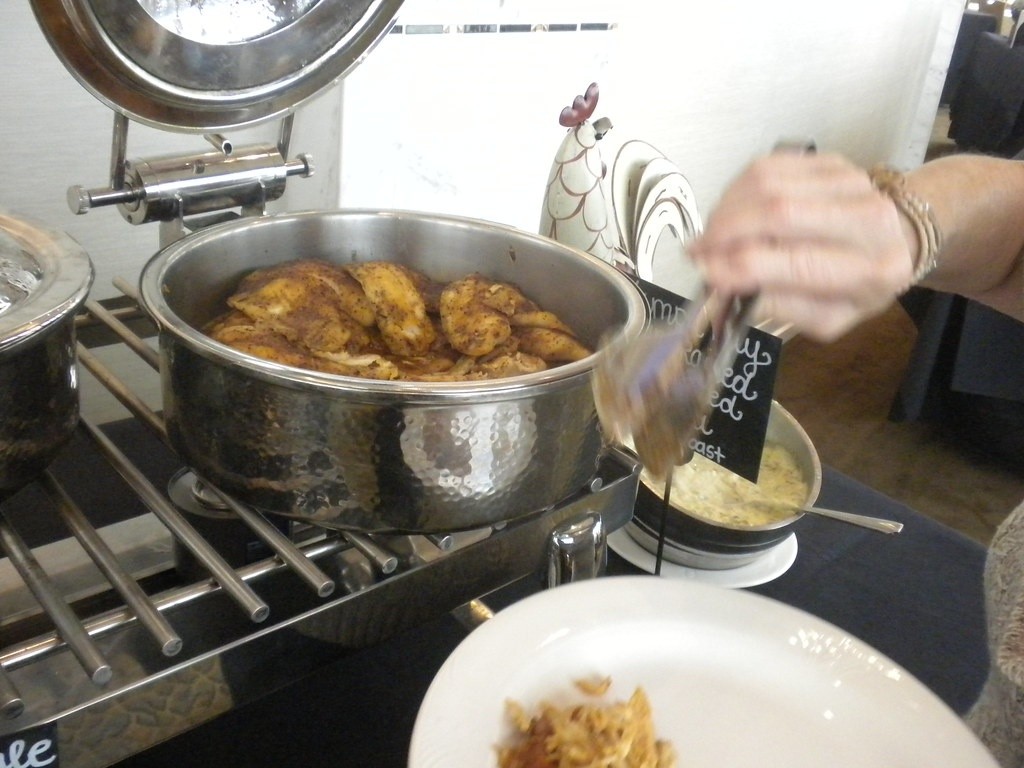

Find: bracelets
869;162;941;286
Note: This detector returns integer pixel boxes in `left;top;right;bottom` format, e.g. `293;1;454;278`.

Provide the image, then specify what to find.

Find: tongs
593;139;821;472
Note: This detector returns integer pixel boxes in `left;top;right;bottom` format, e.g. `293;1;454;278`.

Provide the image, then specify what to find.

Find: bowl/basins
628;397;823;567
0;208;93;502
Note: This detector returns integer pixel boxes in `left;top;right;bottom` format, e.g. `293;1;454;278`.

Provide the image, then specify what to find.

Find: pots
30;0;650;534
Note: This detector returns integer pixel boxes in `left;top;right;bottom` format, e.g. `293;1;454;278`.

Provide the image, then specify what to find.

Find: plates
406;577;1000;768
607;520;797;587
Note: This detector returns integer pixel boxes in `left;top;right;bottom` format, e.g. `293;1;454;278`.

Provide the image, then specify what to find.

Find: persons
696;151;1024;768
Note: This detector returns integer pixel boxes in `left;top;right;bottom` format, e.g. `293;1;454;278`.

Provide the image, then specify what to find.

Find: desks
0;412;991;768
